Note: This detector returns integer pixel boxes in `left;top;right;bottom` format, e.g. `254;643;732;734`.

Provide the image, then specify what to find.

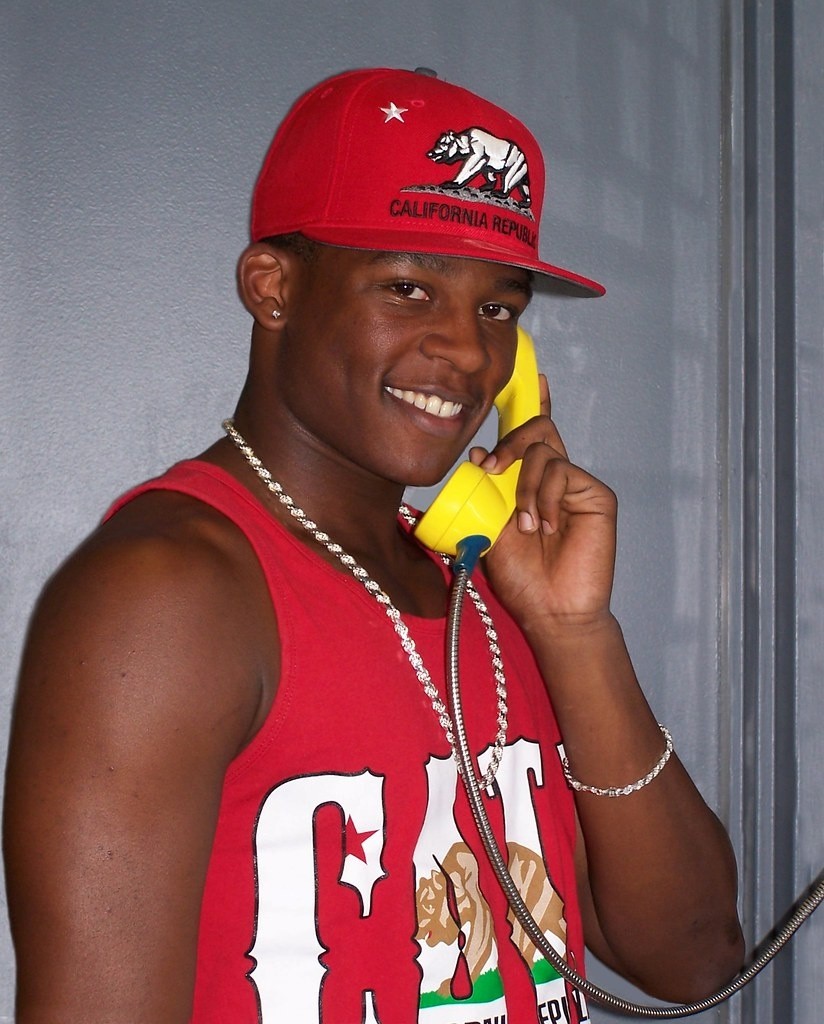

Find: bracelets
563;723;675;798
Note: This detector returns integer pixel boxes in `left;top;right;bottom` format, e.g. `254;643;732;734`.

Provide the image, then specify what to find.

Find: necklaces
221;418;507;792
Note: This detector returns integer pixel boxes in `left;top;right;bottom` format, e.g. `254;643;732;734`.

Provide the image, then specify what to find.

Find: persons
0;65;747;1024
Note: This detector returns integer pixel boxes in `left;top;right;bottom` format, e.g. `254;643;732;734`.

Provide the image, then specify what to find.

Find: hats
250;68;606;298
